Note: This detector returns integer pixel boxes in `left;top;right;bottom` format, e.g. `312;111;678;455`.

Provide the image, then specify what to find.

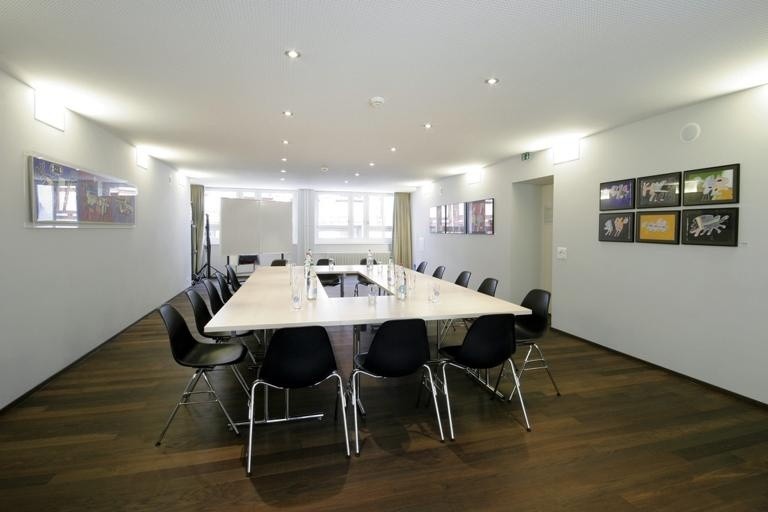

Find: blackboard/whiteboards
221;198;292;256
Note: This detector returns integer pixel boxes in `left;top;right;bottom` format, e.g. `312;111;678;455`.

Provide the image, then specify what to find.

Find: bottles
304;248;409;303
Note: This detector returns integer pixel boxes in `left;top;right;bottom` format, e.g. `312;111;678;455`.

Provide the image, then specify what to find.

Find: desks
202;265;532;422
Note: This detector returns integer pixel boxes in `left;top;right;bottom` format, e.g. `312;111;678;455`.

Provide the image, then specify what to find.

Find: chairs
247;325;350;474
181;287;260;400
350;318;445;457
316;259;344;297
491;288;561;402
270;259;289;266
354;259;383;295
436;314;532;441
156;303;251;446
415;261;427;273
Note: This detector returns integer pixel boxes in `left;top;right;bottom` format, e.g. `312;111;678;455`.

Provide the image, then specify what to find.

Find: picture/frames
428;198;495;237
597;162;740;251
27;154;139;230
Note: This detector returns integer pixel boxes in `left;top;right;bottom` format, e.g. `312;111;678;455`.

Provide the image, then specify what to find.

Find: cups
289;262;303;309
425;282;440;303
409;272;416;289
